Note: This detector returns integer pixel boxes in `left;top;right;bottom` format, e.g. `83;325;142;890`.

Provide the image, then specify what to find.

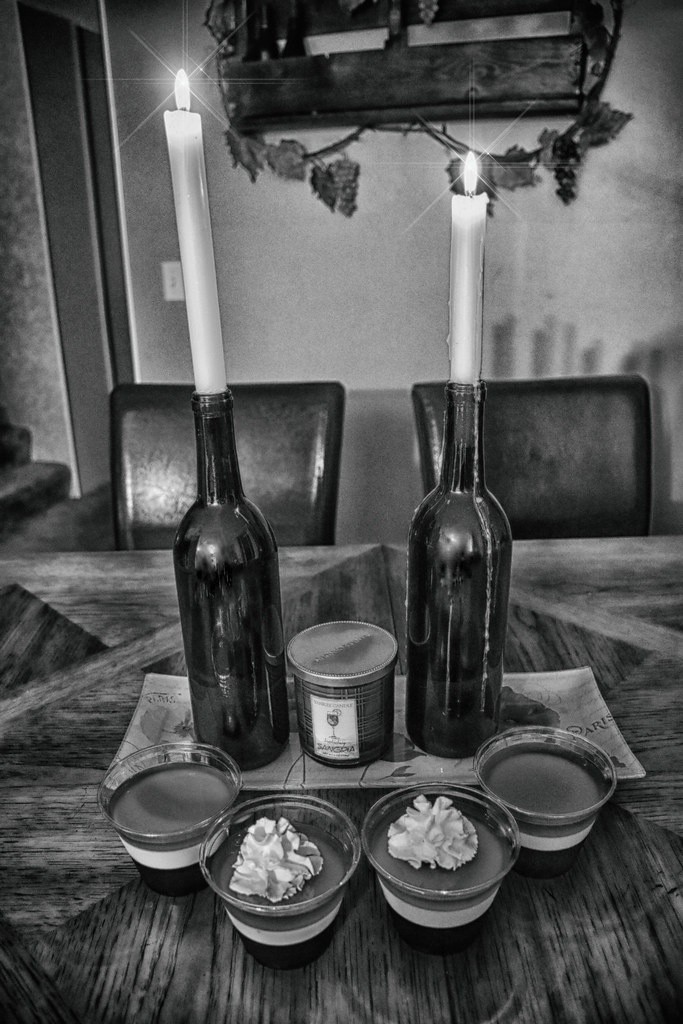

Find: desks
0;536;683;1024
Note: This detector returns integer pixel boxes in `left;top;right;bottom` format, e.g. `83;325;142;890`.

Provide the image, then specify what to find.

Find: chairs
411;374;654;542
109;384;341;551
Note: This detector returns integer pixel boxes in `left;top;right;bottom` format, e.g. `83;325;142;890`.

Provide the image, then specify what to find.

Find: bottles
173;388;289;771
406;380;512;761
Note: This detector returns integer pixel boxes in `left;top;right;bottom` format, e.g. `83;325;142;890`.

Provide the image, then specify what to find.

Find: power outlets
160;260;185;302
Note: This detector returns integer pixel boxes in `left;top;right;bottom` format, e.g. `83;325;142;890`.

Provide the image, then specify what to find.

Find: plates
104;667;646;790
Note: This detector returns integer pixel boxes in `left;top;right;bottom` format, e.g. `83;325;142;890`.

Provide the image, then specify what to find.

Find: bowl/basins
97;742;243;896
361;783;521;929
474;726;617;852
199;794;361;946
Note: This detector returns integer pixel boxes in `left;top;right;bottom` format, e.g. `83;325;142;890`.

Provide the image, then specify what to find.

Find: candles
165;69;229;395
447;151;488;388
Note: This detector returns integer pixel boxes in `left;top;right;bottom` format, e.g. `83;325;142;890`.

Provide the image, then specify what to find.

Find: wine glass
326;712;339;739
